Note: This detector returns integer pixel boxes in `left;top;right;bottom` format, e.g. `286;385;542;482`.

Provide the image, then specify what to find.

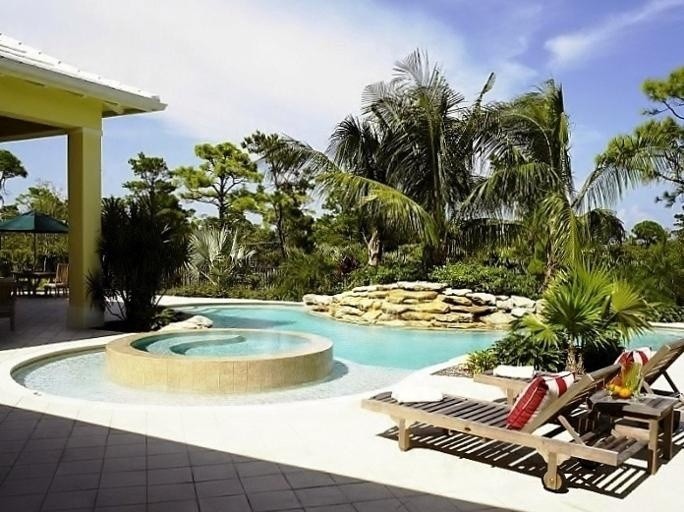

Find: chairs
0;256;70;331
474;338;684;475
361;364;651;492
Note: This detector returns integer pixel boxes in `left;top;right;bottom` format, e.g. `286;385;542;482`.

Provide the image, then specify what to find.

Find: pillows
505;371;576;431
609;346;653;387
